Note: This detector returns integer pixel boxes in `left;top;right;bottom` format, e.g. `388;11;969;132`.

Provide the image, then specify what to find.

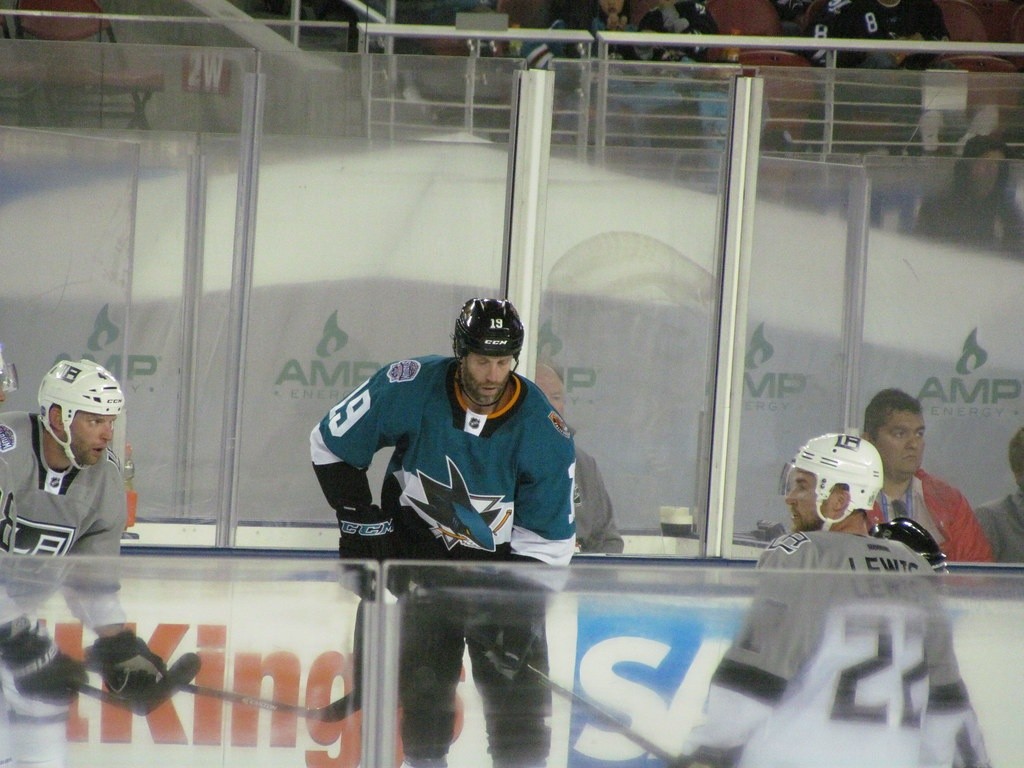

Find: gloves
84;628;167;703
466;569;553;681
334;504;409;602
667;744;747;768
0;614;89;706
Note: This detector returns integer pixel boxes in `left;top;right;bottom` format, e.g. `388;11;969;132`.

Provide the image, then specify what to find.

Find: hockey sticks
56;651;204;718
71;655;362;724
425;601;689;766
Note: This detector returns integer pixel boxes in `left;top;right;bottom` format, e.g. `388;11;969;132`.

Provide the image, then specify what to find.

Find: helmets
0;343;18;393
777;433;883;523
454;298;524;359
38;359;125;431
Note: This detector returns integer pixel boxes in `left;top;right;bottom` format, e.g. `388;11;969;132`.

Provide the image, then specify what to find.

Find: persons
531;357;625;556
679;432;991;768
309;296;576;768
972;427;1024;563
0;359;167;768
859;389;1000;562
